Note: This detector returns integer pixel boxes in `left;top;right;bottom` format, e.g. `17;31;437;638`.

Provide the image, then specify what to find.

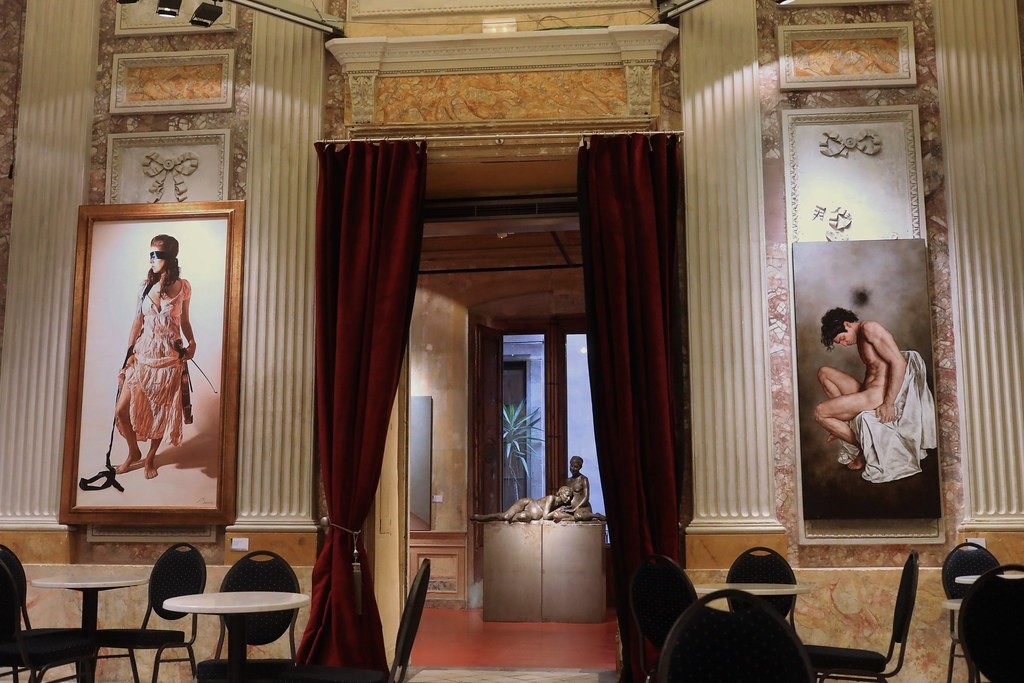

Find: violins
174;338;193;425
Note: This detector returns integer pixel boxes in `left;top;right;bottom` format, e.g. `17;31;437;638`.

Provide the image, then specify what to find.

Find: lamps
189;0;223;27
155;0;183;19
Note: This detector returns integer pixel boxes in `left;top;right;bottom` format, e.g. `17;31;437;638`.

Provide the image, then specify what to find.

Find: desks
694;583;812;596
941;599;964;610
955;574;1024;584
162;591;309;683
30;573;150;683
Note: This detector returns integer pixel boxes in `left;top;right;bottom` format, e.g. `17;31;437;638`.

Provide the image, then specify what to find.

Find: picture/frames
57;199;246;526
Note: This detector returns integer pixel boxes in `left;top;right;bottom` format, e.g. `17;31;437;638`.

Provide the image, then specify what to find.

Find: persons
469;454;613;523
811;305;909;471
112;231;197;479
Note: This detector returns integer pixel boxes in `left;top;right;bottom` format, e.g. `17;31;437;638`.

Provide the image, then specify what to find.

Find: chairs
656;588;814;683
95;543;207;682
725;546;797;634
0;543;103;683
958;563;1024;683
197;550;309;683
299;557;431;683
802;550;919;682
628;554;699;683
942;542;1004;682
